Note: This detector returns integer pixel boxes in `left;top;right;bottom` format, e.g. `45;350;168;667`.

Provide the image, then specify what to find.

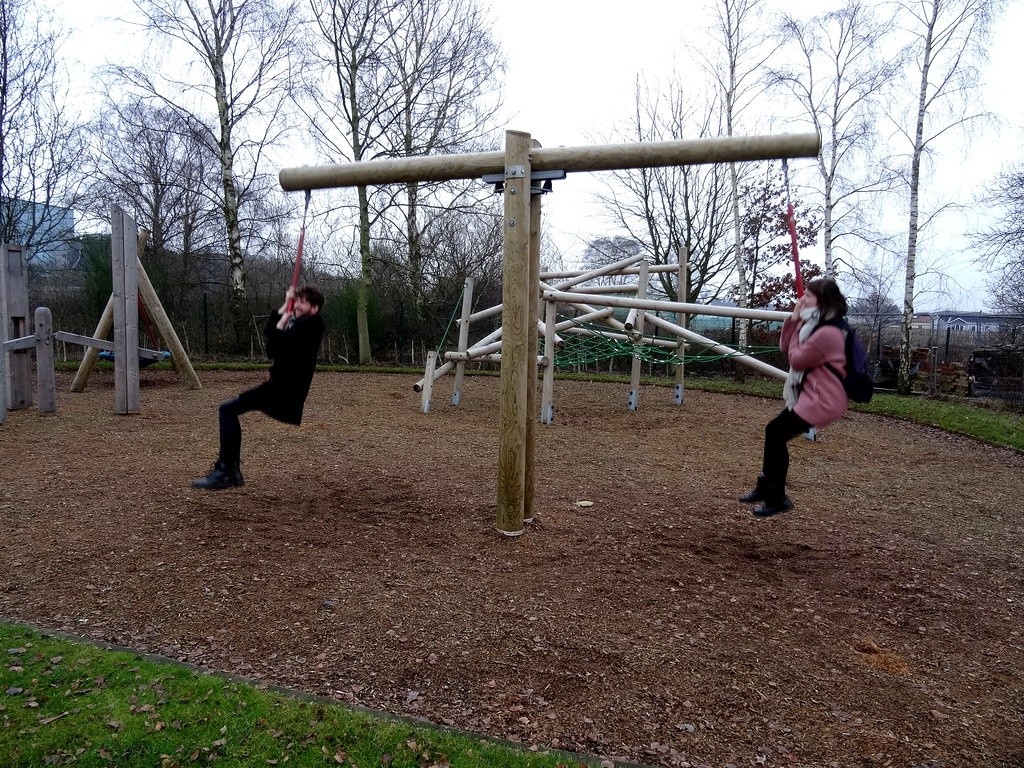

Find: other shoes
192;462;244;490
740;475;770;503
753;495;793;517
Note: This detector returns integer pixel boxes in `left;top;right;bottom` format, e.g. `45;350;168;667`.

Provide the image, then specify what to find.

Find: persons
192;286;324;489
740;279;848;516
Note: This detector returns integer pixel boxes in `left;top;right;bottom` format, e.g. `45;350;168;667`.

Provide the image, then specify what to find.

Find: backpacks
809;317;873;404
